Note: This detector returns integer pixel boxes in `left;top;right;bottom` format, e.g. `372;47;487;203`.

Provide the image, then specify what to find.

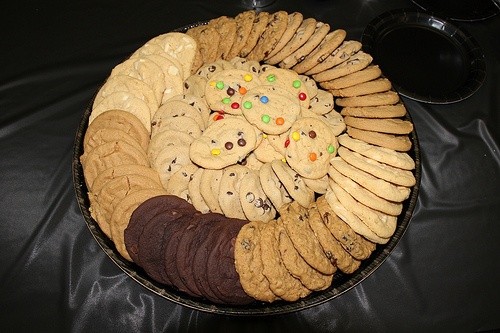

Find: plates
411;0;500;21
71;13;421;316
361;11;487;103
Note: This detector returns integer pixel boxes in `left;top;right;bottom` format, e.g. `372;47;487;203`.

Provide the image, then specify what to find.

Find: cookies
79;10;415;302
